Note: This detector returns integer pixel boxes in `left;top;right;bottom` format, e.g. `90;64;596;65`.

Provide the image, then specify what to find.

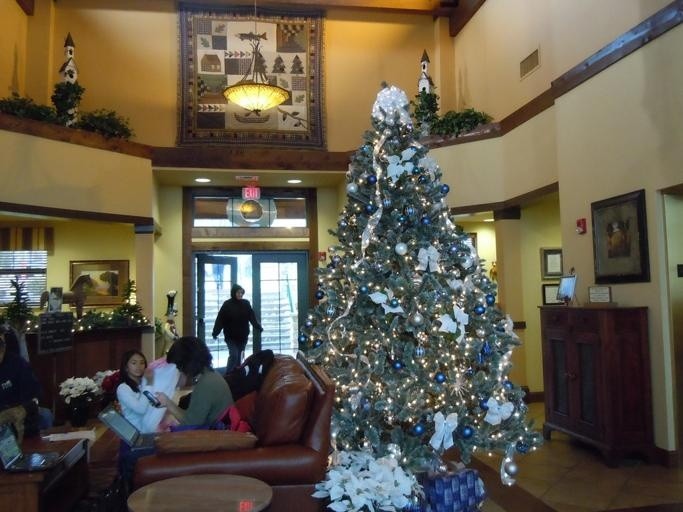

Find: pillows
155;428;257;453
254;353;313;447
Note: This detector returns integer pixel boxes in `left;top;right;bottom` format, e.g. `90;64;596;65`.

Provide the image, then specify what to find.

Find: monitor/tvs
556;274;578;302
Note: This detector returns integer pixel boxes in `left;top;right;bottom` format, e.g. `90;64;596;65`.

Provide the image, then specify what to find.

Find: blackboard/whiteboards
38;312;74;355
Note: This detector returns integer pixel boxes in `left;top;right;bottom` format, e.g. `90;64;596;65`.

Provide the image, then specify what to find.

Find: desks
126;473;273;512
538;307;653;469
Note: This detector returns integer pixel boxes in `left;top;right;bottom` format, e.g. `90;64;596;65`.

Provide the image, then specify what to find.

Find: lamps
224;2;292;114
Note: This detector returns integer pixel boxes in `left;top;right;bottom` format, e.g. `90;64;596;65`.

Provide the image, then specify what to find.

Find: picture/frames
541;249;563;278
69;260;129;308
543;284;562;304
591;189;651;283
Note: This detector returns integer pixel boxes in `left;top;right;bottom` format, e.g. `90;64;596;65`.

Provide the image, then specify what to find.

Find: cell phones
143;390;160;406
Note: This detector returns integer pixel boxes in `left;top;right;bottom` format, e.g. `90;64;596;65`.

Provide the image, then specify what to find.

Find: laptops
98;404;163;451
0;422;61;472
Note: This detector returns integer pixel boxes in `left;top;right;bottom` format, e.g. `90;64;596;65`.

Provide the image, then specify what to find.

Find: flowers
60;377;101;404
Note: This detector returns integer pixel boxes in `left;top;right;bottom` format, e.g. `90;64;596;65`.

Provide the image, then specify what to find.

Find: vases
67;395;93;427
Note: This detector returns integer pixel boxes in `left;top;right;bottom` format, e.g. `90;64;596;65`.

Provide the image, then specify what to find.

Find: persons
149;336;235;431
112;347;181;434
0;334;42;439
212;284;263;373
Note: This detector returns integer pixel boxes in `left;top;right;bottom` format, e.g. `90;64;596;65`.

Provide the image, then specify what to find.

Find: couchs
133;349;335;512
